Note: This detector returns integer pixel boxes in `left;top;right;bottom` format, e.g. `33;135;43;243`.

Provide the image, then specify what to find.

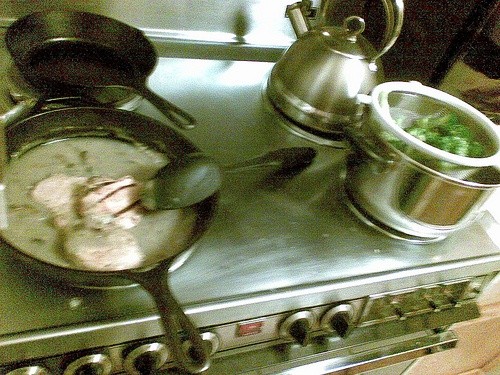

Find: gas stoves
0;42;500;374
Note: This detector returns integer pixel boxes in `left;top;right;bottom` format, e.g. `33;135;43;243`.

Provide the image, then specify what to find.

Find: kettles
265;0;404;136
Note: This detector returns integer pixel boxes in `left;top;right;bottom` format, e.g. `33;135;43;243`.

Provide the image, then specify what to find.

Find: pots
5;9;198;130
341;80;500;239
1;106;223;373
